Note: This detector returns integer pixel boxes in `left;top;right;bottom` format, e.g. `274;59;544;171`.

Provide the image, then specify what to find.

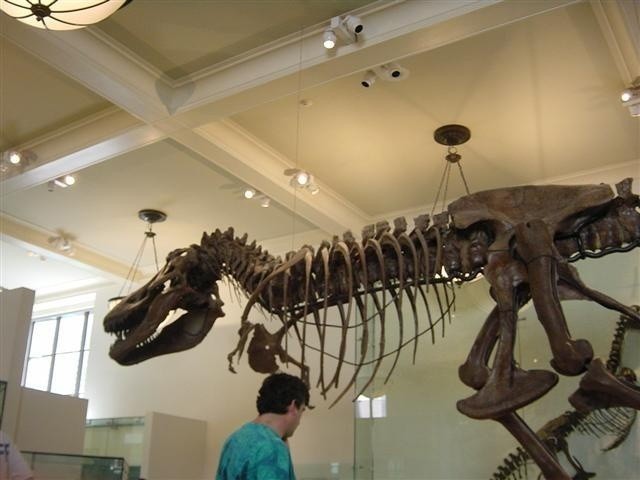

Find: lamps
0;149;24;168
241;187;256;200
107;209;175;344
0;0;135;32
321;15;400;88
286;168;319;196
419;122;489;285
260;197;270;208
620;88;640;118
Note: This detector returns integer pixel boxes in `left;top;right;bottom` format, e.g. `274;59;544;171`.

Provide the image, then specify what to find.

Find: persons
213;373;312;480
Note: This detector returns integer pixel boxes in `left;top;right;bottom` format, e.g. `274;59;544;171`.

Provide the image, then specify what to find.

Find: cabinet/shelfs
16;385;206;480
